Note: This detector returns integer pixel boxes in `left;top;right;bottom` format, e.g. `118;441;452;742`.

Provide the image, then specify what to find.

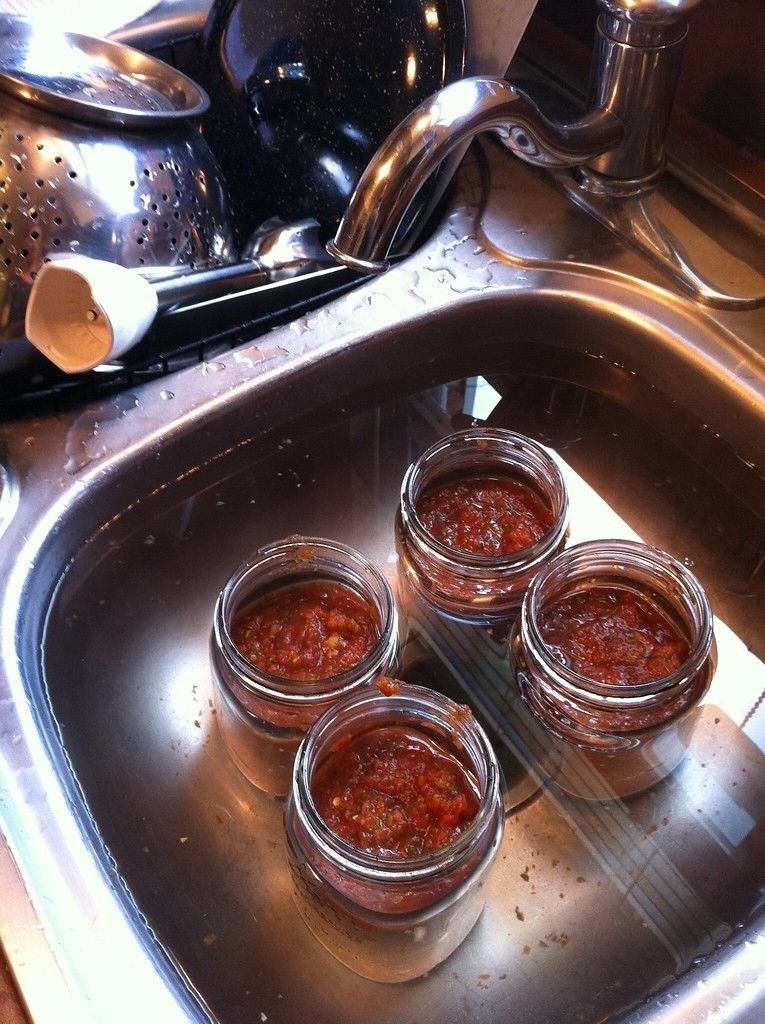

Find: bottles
512;539;718;801
394;430;568;665
208;534;402;796
284;681;505;985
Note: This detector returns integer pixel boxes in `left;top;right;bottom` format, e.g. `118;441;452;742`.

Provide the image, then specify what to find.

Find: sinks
0;1;473;433
0;282;765;1024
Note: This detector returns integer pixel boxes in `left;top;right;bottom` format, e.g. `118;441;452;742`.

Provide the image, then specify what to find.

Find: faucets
325;1;706;276
